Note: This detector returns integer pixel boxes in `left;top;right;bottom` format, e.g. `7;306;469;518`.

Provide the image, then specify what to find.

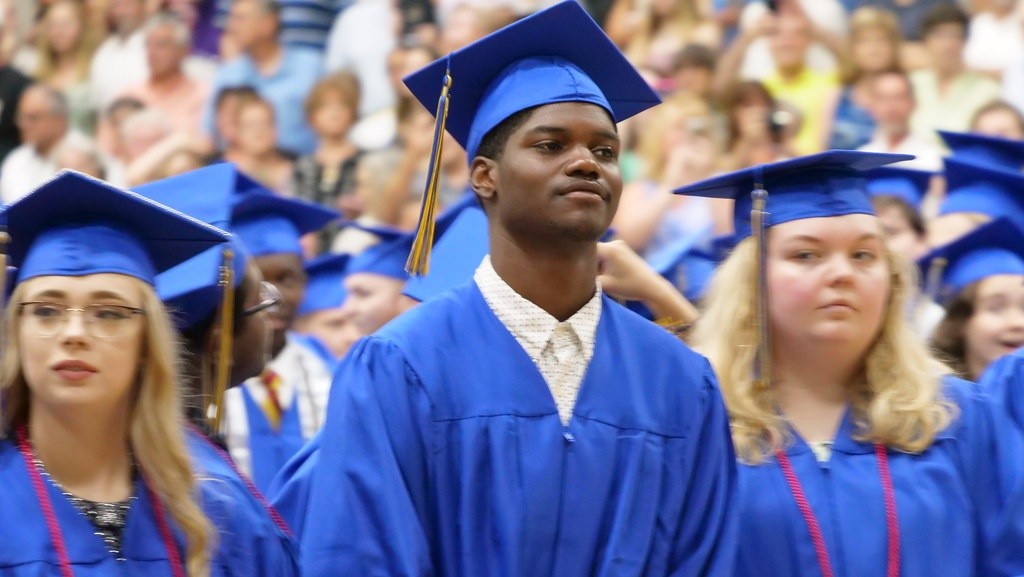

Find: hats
130;129;1024;332
403;0;662;278
0;169;233;438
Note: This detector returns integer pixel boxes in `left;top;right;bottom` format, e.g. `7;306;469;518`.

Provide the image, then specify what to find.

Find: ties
261;372;286;431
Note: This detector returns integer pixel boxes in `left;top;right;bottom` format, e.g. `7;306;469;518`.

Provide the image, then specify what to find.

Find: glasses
235;280;284;321
17;300;146;337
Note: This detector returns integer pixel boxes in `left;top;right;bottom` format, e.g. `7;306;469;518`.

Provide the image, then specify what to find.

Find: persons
0;0;1024;577
